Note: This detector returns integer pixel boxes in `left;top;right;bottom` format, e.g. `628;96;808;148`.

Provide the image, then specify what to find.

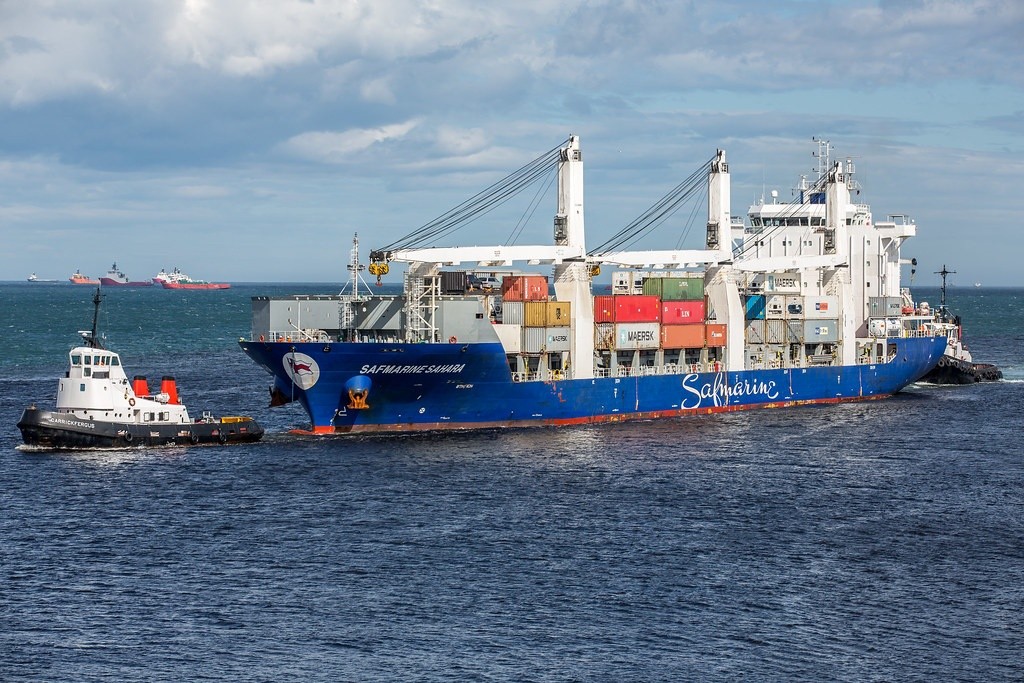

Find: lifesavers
449;335;457;344
938;358;1003;381
219;431;228;443
125;431;133;442
129;397;136;406
259;335;264;342
191;433;200;445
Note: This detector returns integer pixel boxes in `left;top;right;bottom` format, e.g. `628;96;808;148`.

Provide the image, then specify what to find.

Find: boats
27;271;58;283
152;266;231;289
17;286;265;453
68;267;101;284
900;265;1002;383
238;131;948;437
99;261;156;286
